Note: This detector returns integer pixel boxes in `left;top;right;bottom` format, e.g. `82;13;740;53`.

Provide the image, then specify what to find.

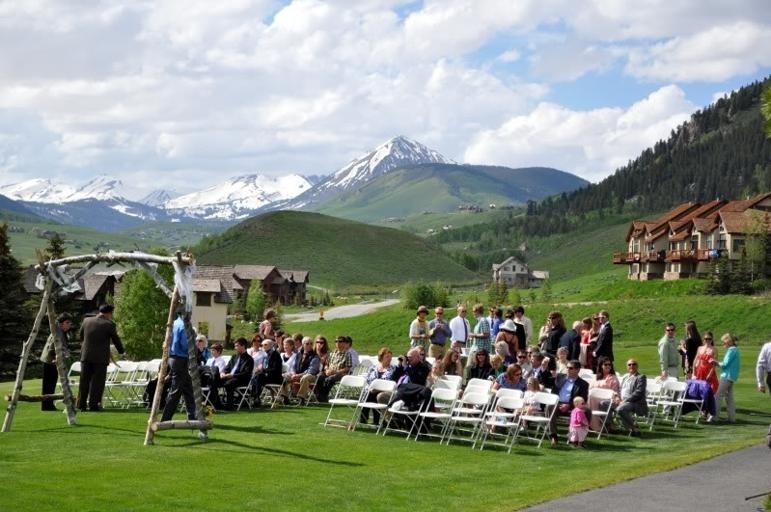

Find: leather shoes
550;436;557;446
81;407;100;411
277;398;290;405
295;402;306;406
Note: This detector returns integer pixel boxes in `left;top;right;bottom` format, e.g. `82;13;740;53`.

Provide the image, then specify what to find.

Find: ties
462;319;468;342
211;359;216;366
490;319;494;329
230;354;241;376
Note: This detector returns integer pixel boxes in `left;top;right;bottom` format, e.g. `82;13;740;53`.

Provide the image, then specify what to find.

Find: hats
100;304;114;312
416;305;429;314
499;319;517;332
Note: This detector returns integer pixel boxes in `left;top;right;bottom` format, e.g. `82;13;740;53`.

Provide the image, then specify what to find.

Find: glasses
477;354;485;355
549;316;556;320
593;316;608;320
567;367;573;370
601;363;611;366
316;342;324;343
627;364;637;366
704;338;712;340
665;329;674;331
335;341;342;342
518;356;526;358
304;343;312;344
420;354;424;355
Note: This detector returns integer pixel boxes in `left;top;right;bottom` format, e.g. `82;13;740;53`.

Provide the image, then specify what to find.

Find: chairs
52;355;322;414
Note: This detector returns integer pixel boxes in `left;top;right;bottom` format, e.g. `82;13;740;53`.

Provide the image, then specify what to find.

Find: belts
452;339;466;344
431;342;445;346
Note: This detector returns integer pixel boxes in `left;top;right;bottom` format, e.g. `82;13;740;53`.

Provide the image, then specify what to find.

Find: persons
39;312;73;411
657;320;740;427
600;358;649;433
358;303;621;450
142;307;359;411
76;303;126;410
160;306;196;421
756;341;771;448
